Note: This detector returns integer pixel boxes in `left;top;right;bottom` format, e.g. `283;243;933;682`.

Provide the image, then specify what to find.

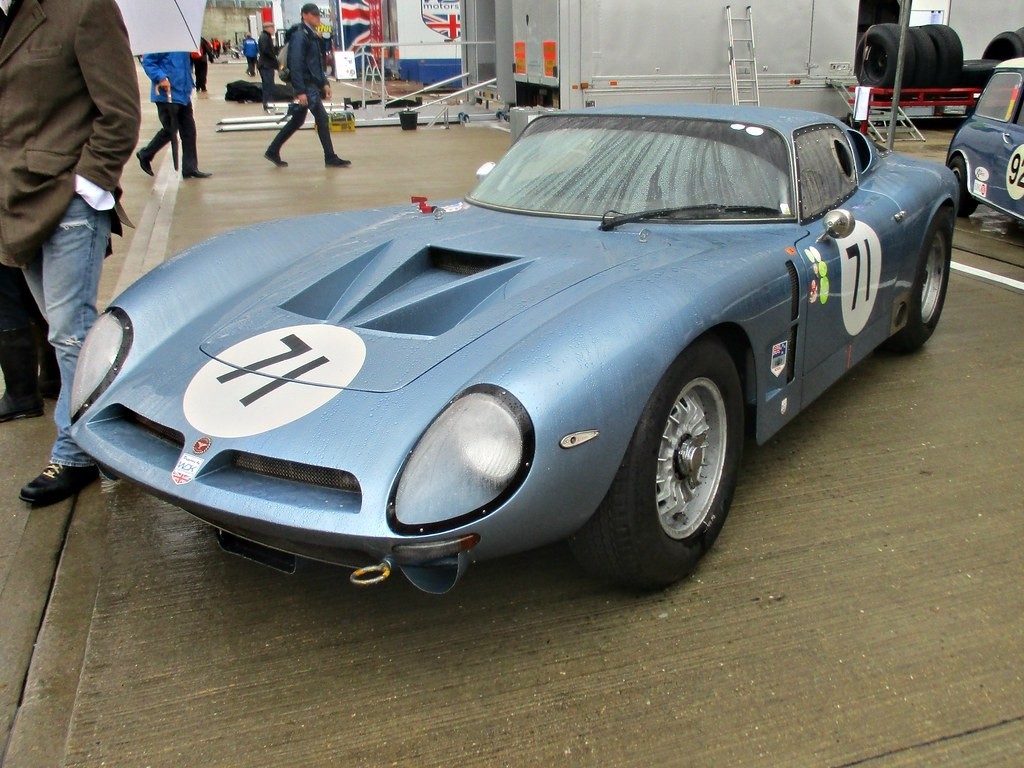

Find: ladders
726;4;760;107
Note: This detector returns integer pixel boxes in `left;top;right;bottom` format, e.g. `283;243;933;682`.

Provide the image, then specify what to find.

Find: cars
942;52;1024;225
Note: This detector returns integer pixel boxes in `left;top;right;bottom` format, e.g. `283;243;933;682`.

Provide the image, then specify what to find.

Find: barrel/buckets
398;110;419;130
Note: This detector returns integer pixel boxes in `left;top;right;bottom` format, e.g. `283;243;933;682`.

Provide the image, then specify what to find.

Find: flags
340;0;382;65
423;14;462;39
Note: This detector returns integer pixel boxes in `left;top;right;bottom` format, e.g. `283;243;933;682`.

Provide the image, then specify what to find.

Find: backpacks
277;41;291;82
191;45;203;59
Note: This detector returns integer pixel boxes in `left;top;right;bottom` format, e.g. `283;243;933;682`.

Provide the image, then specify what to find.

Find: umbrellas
155;81;179;171
115;0;208;56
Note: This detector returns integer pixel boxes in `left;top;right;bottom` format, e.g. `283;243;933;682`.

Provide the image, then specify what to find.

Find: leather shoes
136;152;154;177
182;168;212;178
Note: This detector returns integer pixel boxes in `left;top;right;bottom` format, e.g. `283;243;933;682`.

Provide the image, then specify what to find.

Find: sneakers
19;462;99;503
0;391;44;422
98;463;120;482
325;154;351;168
39;374;60;400
264;145;288;167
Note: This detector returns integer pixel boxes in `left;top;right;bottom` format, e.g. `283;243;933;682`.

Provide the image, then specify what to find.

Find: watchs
165;77;168;81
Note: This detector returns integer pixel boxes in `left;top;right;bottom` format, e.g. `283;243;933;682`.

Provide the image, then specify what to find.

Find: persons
190;36;231;92
136;52;212;178
0;0;141;506
242;3;351;168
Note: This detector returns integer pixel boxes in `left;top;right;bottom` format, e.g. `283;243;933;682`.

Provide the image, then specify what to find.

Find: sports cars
67;99;960;597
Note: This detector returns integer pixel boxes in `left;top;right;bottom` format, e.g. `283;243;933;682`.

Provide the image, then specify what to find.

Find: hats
302;3;323;13
263;23;275;28
